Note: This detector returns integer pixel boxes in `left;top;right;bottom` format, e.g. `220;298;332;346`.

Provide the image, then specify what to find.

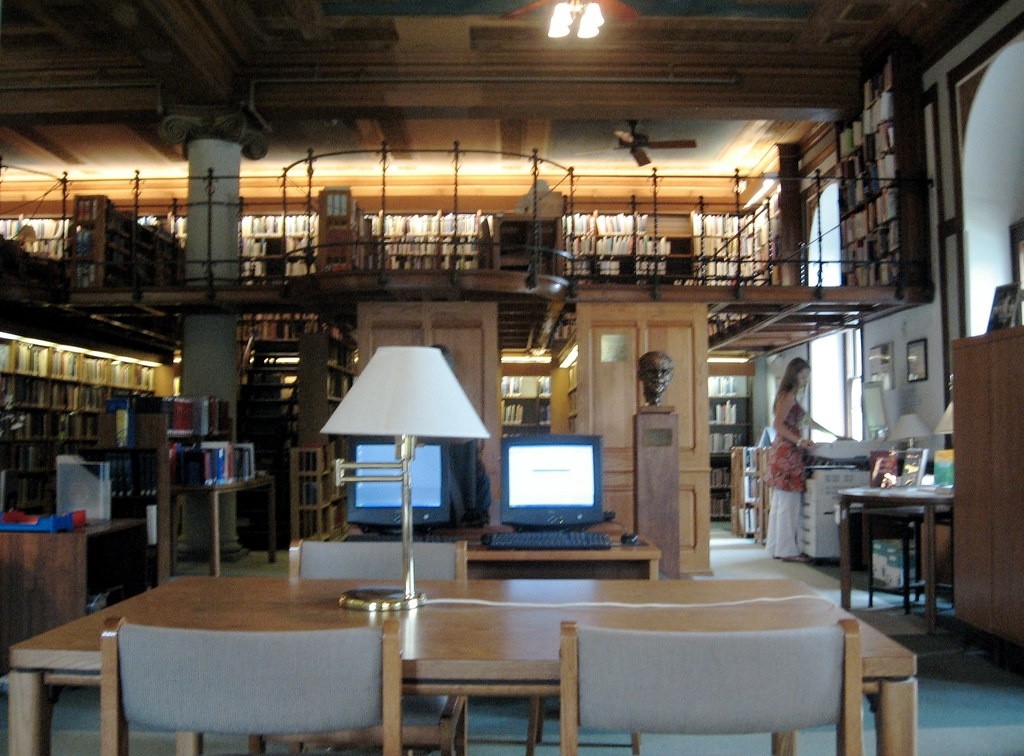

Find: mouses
621;534;638;546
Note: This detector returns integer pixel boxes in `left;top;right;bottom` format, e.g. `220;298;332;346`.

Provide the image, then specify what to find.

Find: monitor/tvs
499;434;604;531
346;438;450;537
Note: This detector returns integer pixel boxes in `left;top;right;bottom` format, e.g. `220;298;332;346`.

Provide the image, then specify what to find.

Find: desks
154;472;281;578
339;523;662;578
832;486;954;632
8;579;918;756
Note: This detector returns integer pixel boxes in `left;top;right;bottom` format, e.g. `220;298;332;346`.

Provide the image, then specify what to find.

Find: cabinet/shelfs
297;332;353;538
0;187;648;275
709;396;749;518
688;203;777;286
0;331;173;513
552;345;586;432
501;356;551;437
826;52;926;288
729;445;777;544
0;507;156;689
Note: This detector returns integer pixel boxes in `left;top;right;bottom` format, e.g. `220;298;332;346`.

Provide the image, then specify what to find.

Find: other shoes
783;556;806;563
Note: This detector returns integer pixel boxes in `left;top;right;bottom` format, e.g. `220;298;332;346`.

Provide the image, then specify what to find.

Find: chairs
558;616;864;755
248;540;470;756
93;617;407;756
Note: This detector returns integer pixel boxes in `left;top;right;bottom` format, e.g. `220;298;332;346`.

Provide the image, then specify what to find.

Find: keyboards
345;535;456;543
488;532;612;549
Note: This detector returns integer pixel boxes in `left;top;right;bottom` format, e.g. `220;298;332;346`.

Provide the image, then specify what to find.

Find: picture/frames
906;337;928;382
987;282;1019;329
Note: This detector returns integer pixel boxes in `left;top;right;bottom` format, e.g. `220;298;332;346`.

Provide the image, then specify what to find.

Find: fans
579;117;697;167
499;0;639;23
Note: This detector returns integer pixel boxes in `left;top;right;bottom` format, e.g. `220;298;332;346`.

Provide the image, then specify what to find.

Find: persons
429;344;491;525
763;357;851;562
638;351;674;407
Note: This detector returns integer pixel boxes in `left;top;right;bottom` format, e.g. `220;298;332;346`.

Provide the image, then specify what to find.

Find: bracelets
796;438;803;446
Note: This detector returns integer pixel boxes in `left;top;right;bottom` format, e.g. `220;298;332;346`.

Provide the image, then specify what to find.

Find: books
562;210;671;274
838;56;900;285
692;192;779;285
364;214;494;269
242;214;319;275
0;215;188;287
501;376;550;437
870;449;954;493
0;343;253;510
708;376;758;532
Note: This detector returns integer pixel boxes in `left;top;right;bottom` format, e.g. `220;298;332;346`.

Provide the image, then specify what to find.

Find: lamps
934;404;953;435
547;1;604;39
318;344;488;610
887;413;931;449
